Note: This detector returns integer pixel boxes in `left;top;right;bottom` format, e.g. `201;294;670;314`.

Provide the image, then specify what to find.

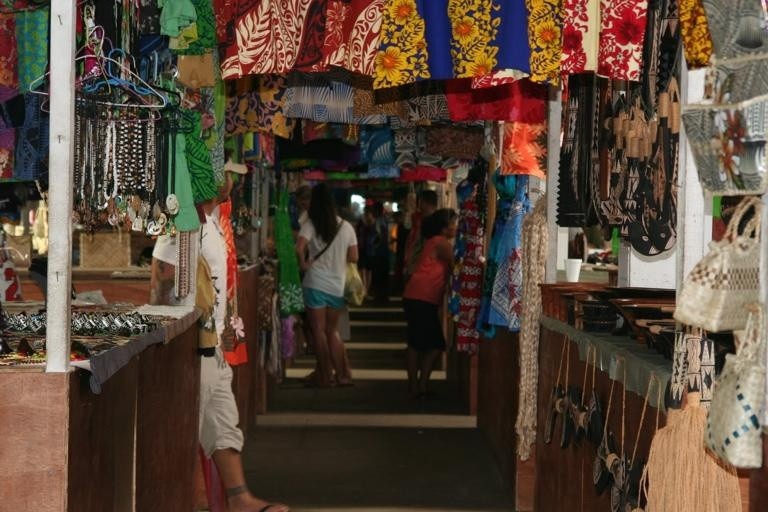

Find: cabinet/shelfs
534;314;673;512
0;264;258;512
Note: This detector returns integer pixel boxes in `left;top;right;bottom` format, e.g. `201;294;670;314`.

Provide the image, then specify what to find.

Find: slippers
303;375;358;389
241;501;294;511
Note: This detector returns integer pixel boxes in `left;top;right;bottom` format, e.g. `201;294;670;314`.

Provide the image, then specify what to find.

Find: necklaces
72;90;180;238
174;231;194;300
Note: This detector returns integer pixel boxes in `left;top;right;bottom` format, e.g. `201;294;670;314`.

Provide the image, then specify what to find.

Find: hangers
30;27;194;136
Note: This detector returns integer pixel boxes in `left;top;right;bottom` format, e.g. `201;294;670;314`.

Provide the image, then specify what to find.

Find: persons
149;152;291;512
293;182;460;402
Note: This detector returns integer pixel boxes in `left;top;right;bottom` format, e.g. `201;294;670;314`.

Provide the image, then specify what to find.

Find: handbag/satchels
671;92;767;469
543;387;637;508
